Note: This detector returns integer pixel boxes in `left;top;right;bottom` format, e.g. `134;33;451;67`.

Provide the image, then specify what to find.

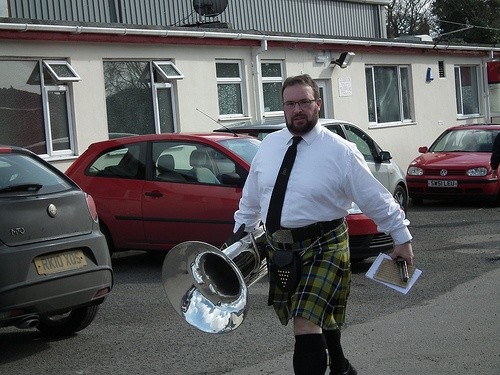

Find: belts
265;136;302;235
264;217;344;244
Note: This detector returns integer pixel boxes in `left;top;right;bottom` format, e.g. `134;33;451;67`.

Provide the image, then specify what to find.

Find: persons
232;76;414;375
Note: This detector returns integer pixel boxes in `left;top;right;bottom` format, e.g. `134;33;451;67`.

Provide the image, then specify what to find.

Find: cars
1;144;115;343
63;131;397;267
212;116;409;213
405;123;500;209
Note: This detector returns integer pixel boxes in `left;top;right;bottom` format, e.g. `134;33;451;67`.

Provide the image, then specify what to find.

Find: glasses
284;98;317;111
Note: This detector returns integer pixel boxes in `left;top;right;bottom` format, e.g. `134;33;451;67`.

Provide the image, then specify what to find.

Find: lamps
330;52;355;69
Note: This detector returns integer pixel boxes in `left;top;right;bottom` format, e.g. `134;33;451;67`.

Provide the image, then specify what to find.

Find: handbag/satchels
272;250;302;293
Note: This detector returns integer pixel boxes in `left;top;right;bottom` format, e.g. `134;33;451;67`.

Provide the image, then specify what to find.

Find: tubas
161;224;269;335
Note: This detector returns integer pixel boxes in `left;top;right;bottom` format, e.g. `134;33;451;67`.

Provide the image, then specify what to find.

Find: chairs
462;134;480;151
189;149;221;184
157;155;188;183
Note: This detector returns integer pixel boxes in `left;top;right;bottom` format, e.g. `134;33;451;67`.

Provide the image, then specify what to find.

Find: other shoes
328;358;358;375
